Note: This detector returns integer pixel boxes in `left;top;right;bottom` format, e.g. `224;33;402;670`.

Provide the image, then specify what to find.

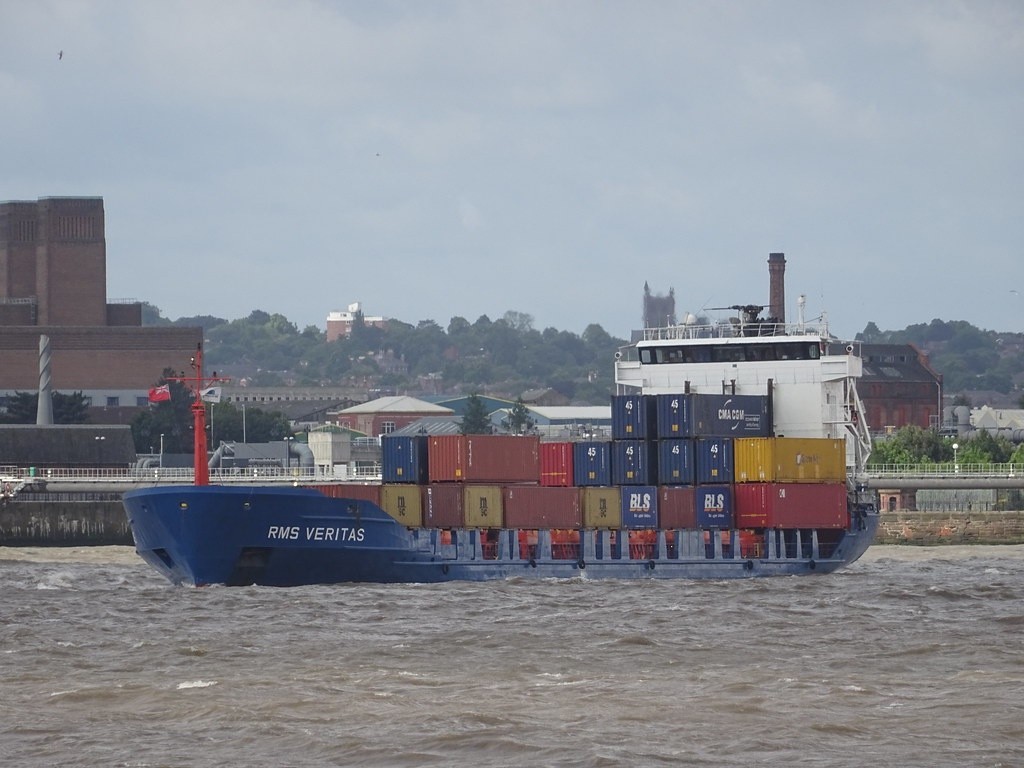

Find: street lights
952;443;959;463
210;403;215;449
241;404;246;443
284;436;293;476
159;434;164;454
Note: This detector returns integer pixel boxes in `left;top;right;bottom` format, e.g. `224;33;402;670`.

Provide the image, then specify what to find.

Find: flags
148;383;171;402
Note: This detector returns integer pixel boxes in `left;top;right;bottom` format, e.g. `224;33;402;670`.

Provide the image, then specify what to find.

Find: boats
118;250;882;588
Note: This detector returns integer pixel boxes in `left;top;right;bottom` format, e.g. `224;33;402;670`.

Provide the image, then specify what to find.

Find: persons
4;481;11;493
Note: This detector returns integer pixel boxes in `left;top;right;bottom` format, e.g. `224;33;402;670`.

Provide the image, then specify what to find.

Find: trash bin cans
889;497;896;511
